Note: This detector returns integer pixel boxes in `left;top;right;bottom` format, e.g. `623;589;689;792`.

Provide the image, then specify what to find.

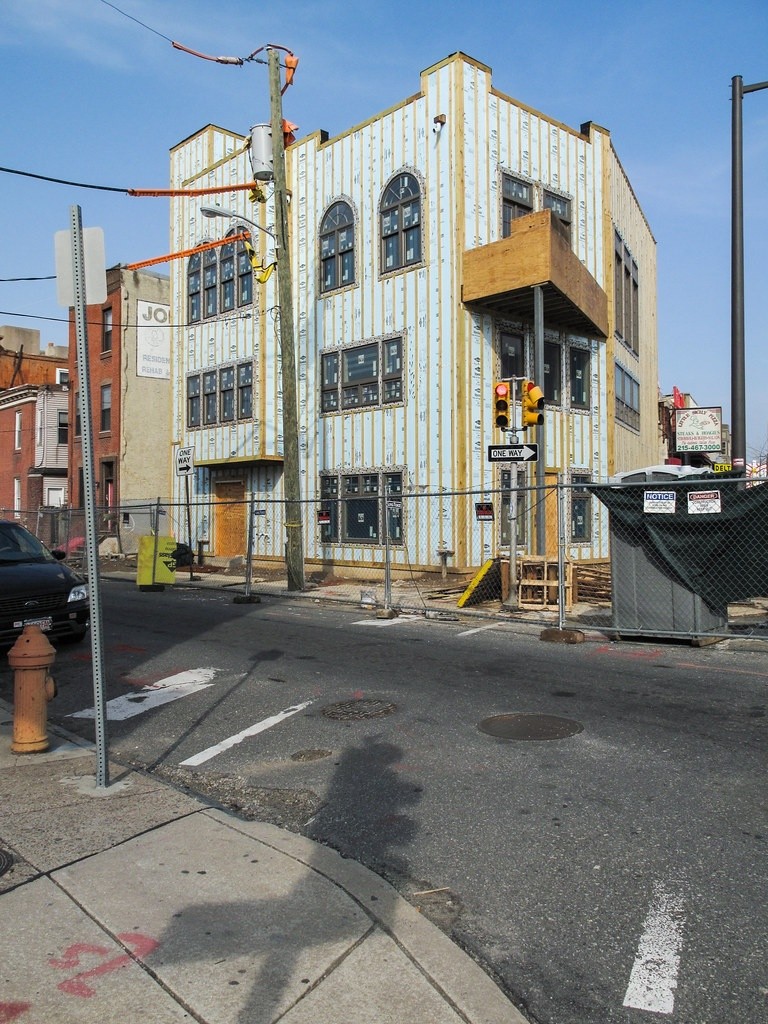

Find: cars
0;517;90;655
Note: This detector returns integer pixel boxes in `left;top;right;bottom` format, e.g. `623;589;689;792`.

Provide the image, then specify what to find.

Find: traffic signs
488;443;540;464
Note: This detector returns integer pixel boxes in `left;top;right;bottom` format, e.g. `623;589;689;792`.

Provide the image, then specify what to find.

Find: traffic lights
495;383;510;429
520;379;544;430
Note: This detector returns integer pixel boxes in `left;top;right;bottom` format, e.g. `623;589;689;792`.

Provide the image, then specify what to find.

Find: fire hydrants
8;626;57;757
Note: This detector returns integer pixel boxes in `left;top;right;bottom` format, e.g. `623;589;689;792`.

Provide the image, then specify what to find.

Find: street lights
199;205;305;591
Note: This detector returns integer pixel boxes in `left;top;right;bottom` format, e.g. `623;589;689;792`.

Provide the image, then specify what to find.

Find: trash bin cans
608;465;745;648
